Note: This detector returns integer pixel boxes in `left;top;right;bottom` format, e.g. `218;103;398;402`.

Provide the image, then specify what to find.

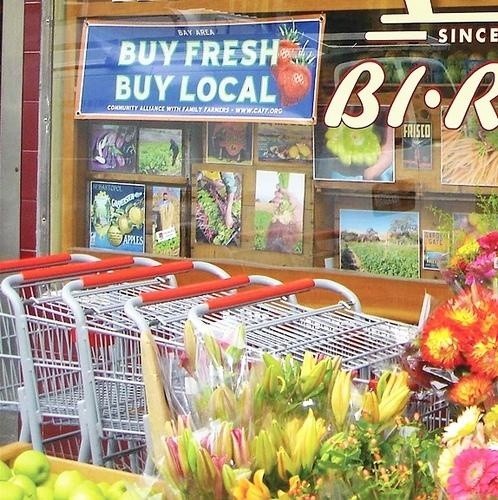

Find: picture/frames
205;121;254;166
89;181;146;253
422;229;450;270
401;121;433;170
339;208;420;279
440;104;498;188
73;13;327;125
451;212;473;257
312;105;396;183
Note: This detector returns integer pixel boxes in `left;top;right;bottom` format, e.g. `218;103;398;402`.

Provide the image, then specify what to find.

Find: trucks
303;29;477;241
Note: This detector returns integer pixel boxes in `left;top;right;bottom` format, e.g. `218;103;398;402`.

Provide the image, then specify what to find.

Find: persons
157;193;169;207
344;106;393;180
169;139;179;167
273;188;303;227
220;172;238;228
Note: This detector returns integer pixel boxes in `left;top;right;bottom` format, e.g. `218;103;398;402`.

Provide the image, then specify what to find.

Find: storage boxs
0;443;166;500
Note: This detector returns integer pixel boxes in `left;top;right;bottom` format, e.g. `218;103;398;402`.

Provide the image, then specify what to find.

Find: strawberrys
276;39;317;108
270;18;305;79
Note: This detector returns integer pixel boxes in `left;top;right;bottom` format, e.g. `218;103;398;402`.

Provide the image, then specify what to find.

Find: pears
119;215;132;235
129;206;144;226
106;224;124;247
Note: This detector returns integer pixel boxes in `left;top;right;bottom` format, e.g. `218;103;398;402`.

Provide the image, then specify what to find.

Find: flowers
141;183;498;500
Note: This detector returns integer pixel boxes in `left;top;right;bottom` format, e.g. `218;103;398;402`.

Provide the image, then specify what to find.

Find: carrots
441;107;498;187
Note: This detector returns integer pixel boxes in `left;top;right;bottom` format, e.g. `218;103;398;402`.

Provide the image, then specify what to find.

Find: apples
0;450;144;500
